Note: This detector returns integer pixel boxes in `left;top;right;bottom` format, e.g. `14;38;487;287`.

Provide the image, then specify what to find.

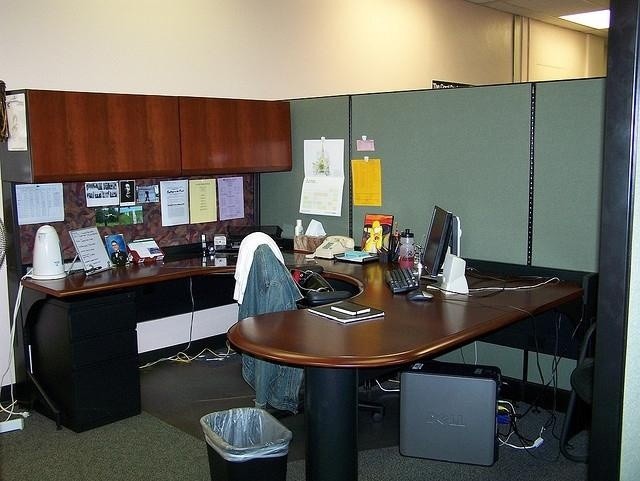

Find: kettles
31;224;66;280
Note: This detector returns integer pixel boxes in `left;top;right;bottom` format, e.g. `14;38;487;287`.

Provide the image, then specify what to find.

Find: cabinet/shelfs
2;88;291;182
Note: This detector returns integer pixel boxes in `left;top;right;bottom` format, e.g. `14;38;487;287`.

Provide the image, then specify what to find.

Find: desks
17;248;585;480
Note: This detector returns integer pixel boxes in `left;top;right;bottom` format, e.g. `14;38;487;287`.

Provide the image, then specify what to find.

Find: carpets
137;344;401;464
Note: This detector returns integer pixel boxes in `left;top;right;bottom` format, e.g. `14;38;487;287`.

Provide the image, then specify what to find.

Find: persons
121;183;134;202
111;240;127;264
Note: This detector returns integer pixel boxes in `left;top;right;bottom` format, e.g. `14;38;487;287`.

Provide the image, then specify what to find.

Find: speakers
440;254;469;294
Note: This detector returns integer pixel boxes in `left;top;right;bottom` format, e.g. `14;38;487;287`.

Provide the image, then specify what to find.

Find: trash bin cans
201;407;293;480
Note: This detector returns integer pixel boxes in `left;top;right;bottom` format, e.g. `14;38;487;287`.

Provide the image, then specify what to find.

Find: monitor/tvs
421;205;462;280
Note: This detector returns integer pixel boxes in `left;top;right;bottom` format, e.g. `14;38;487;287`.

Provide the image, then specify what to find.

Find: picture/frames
70;226;131;276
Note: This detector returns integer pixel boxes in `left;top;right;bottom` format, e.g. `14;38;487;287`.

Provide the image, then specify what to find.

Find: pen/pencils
84;266;102;274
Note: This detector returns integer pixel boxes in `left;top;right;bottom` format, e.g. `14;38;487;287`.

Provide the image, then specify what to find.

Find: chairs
234;232;399;419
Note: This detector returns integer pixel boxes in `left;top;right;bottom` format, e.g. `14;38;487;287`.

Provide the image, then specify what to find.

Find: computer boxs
400;359;501;467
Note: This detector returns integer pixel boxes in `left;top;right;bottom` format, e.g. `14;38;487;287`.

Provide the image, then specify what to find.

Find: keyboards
385;267;419;293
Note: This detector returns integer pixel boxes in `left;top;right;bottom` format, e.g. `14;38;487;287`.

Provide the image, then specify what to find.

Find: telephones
315;236;355;259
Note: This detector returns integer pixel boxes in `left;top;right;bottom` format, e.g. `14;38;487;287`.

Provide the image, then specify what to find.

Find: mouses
407;291;433;300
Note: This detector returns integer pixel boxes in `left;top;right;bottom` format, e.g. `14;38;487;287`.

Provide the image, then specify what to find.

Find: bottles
295;220;303;237
400;229;415;260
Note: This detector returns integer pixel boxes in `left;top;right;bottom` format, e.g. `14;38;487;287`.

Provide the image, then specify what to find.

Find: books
307;300;385;323
